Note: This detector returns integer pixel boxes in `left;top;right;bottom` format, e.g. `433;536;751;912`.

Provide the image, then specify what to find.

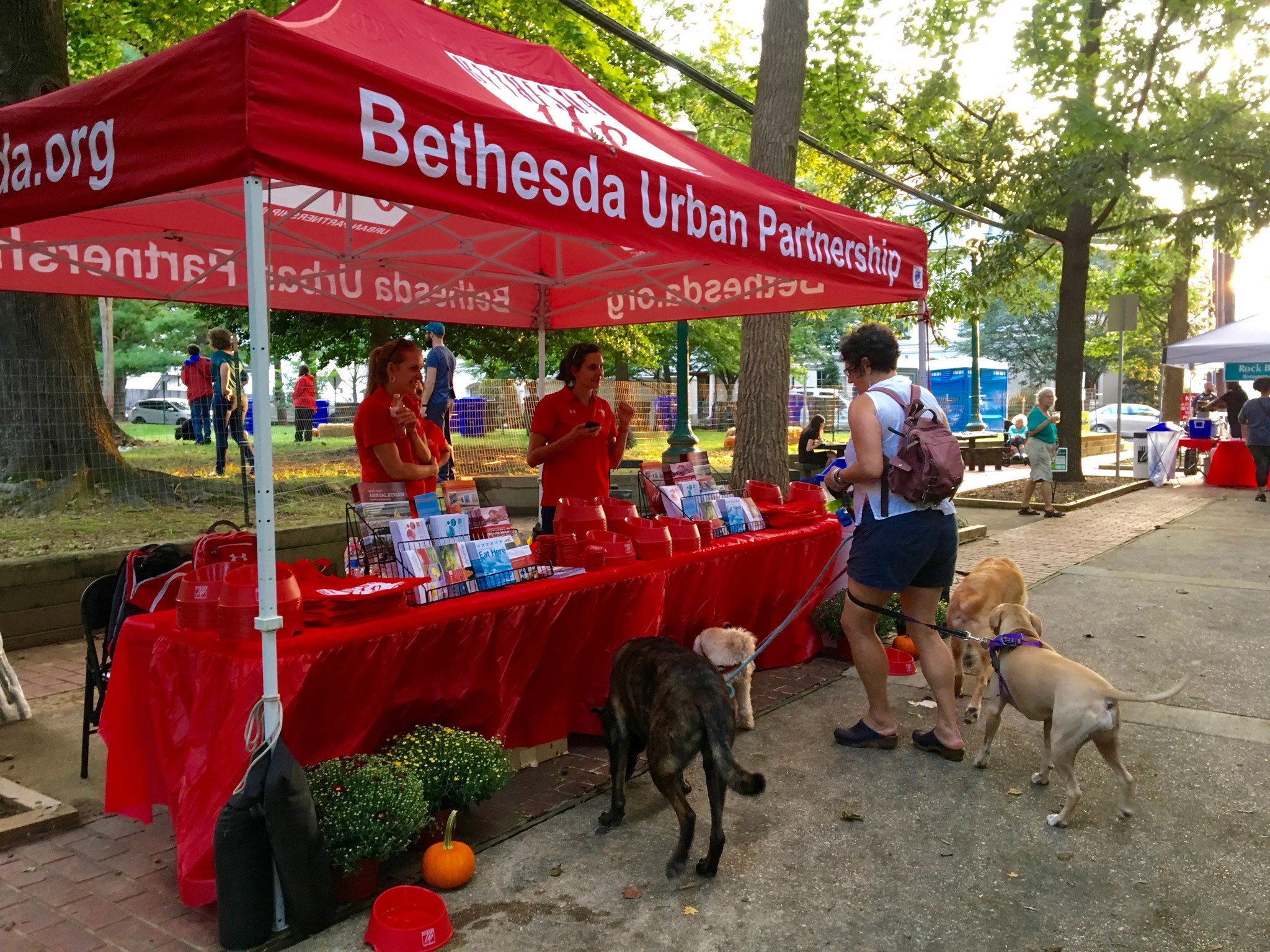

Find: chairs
80;573;119;779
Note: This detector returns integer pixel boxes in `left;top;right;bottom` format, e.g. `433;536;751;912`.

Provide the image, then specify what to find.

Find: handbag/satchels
193;520;258;568
1009;437;1024;447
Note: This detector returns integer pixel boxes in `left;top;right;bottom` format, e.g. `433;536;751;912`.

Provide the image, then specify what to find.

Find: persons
349;338;451;500
180;343;214;445
527;344;635;542
1007;414;1029;460
420;321;457;484
204;329;255;478
1201;382;1248;439
1237;376;1270;502
1190;382;1219;418
291;365;317;442
1019;388;1067;517
824;322;964;765
798;414;836;469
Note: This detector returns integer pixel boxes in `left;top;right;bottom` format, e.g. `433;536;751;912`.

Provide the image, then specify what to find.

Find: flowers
302;722;519;882
812;587;952;641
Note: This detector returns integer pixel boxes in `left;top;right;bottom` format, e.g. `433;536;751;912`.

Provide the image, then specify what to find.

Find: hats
420;321;446;335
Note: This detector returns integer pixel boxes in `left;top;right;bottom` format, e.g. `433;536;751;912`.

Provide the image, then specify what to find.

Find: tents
1157;313;1270;424
0;0;934;935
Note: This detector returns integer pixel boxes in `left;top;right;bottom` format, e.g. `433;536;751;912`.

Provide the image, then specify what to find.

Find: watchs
837;469;847;484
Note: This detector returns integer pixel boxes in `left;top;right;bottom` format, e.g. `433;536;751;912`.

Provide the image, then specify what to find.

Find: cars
128;396;191;426
1088;402;1161;438
789;386;854;433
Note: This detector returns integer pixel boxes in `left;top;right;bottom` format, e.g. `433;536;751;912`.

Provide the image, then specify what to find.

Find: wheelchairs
1002;418;1032;468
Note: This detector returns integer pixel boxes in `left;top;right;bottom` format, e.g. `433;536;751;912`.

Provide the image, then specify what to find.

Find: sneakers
1255;494;1267;502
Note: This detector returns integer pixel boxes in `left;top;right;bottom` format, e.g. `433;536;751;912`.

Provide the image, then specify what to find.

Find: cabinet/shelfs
638;462;767;538
346;502;554;604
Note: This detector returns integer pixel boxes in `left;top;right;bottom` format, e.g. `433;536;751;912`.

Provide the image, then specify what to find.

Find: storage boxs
1188;417;1212;439
503;736;568;772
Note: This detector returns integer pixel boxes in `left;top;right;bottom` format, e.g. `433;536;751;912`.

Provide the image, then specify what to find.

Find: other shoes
1023;456;1031;466
1014;453;1021;460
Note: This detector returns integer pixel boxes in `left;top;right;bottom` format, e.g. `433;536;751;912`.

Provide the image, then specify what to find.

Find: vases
328;857;379;903
417;807;456;850
837;636;855;660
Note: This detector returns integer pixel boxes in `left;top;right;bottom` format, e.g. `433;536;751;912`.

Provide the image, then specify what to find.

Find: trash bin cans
312;399;329;428
455;398;487;437
788;393;803;427
243;399;253;435
1145;421;1184;487
653;396;677;431
450;399;459;433
1133;431;1150;479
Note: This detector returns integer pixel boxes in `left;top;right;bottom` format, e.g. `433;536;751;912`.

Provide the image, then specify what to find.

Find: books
349;480;540;604
639;451;766;540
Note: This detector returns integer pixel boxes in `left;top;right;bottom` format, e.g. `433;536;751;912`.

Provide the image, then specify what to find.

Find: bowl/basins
885;647;918;676
552;495;701;569
362;885;453;952
176;561;305;641
742;479;828;521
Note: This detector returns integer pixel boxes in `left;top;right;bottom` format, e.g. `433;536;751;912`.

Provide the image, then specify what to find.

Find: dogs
591;637;768;878
945;558;1195;825
692;625;757;730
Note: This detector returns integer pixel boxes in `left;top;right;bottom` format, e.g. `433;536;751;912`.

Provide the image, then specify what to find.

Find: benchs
794;461;824;478
959;447;1011;470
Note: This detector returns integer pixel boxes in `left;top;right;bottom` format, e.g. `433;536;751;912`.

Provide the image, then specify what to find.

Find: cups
1051;411;1061;423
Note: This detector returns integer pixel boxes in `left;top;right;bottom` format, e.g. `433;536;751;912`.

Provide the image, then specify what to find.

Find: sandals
1018;506;1040;516
1044;508;1066;518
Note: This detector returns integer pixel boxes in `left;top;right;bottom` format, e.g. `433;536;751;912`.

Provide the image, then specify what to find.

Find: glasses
572;342;600;360
423;331;430;334
384;338;412;368
843;364;854;377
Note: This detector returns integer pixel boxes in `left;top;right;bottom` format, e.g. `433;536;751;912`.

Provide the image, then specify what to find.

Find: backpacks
99;543;194;674
867;385;965;507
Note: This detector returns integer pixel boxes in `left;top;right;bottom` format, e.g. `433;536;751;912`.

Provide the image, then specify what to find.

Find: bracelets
831;468;849;487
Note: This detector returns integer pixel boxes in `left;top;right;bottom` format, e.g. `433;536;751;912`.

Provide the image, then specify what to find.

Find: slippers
912;727;965;762
833;717;899;749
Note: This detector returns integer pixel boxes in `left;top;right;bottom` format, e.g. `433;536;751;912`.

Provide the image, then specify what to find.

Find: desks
1146;429;1184;488
1206;440;1270;489
1167;437;1218;481
98;520;841;907
953;430;998;470
814;443;848;459
